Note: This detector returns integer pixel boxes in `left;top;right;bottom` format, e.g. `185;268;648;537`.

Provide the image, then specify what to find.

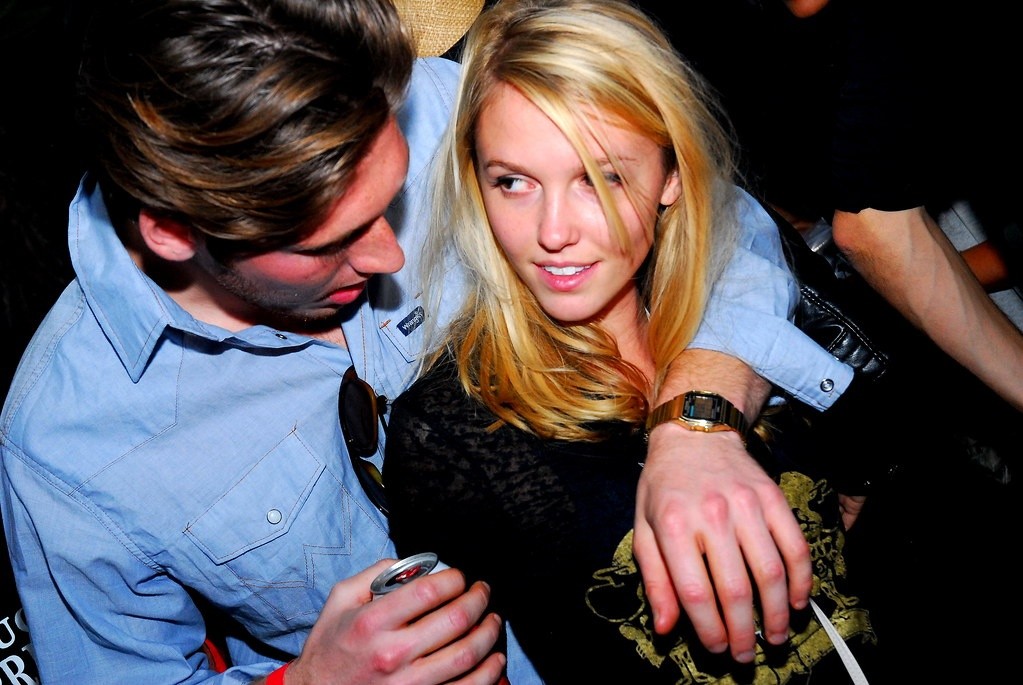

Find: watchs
645;391;748;449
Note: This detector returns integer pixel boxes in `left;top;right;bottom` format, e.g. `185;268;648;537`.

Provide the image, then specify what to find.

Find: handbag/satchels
583;474;875;685
763;283;903;499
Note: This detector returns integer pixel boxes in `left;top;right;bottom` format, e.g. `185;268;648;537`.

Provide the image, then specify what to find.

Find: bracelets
266;659;294;685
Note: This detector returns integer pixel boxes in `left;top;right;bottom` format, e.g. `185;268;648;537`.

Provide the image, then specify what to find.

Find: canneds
370;552;512;685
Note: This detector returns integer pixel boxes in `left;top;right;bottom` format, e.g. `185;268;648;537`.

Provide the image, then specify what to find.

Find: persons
382;0;897;685
831;0;1023;411
1;1;852;685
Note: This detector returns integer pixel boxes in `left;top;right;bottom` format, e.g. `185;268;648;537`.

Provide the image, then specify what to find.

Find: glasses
337;365;388;518
